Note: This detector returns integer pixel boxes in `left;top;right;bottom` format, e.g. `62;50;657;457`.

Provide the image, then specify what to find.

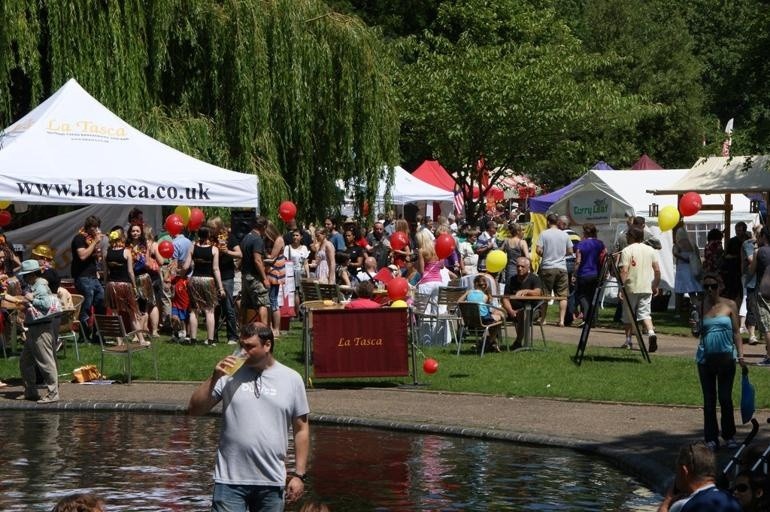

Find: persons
1;205;769;403
190;321;312;511
730;468;769;511
687;275;747;449
51;492;108;512
660;443;744;512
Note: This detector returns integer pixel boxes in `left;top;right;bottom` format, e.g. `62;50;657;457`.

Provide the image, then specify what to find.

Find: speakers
230;209;254;249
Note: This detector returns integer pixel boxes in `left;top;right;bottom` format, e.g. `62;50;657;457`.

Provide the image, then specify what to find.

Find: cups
224;346;250;377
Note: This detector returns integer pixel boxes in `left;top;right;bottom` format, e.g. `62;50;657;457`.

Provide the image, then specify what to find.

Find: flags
453;178;471;214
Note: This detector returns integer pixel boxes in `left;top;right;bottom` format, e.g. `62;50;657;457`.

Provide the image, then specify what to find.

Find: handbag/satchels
74;365;101;383
742;376;754;424
689;254;703;280
696;345;706;365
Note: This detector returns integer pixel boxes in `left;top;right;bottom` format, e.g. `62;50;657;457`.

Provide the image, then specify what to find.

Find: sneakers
477;339;502;352
512;341;522;350
621;342;632;349
132;329;160;348
112;345;128;352
227;340;238;345
273;329;280;337
703;440;718;451
37;398;56;403
740;327;770;366
726;436;738;449
649;334;657;352
169;336;219;348
0;382;7;388
15;394;40;400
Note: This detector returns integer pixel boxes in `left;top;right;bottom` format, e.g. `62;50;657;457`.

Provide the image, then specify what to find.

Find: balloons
0;200;10;210
679;191;703;217
279;201;297;223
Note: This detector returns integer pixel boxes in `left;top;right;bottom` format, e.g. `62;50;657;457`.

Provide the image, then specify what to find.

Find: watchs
294;470;311;486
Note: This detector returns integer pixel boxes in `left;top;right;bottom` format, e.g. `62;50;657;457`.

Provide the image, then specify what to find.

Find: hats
511;202;519;208
17;259;43;275
31;245;55;260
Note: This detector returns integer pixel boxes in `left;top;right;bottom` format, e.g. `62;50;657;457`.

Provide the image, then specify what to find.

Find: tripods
218;250;258;335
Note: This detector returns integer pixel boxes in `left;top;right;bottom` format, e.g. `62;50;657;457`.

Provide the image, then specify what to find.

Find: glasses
703;283;719;290
733;483;750;493
